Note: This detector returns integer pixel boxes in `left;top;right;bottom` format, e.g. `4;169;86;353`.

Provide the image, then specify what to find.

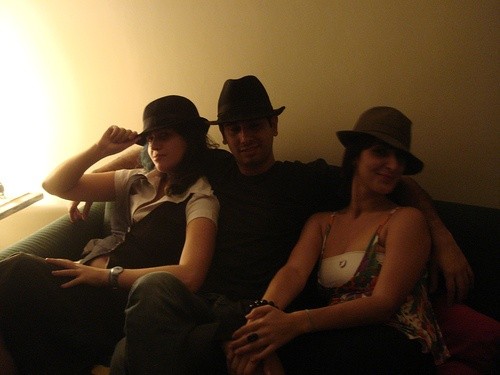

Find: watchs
107;266;124;295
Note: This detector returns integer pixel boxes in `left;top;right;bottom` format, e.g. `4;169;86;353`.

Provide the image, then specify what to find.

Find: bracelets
306;308;314;333
245;299;280;314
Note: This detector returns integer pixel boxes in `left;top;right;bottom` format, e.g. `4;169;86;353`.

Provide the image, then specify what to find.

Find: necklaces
339;248;347;267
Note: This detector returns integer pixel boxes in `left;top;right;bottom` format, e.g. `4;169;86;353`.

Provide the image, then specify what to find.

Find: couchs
0;200;500;375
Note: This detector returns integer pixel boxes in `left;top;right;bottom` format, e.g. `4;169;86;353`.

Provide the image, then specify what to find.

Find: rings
247;331;259;343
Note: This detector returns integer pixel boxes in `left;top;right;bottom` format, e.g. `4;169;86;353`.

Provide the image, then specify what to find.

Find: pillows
432;304;500;375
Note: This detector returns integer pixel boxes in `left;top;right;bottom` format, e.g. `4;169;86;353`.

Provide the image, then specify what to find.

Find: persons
0;95;219;375
211;106;451;375
70;77;474;375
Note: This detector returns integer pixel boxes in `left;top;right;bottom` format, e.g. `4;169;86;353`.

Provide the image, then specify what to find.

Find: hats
208;75;285;125
336;106;424;175
134;95;211;147
